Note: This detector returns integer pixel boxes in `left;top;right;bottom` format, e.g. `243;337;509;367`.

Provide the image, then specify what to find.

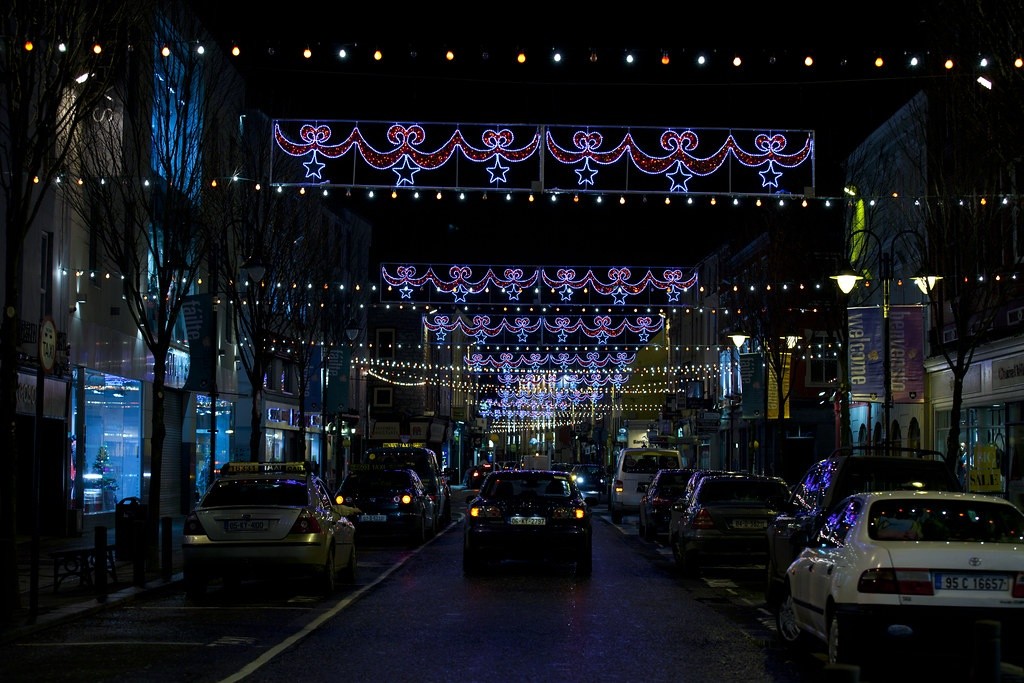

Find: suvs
365;442;453;531
763;444;968;613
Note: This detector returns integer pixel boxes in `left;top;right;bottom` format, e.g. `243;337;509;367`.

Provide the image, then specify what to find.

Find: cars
775;487;1023;667
636;467;712;543
667;470;753;560
332;464;439;544
676;474;795;575
181;461;358;597
463;454;618;495
460;468;593;582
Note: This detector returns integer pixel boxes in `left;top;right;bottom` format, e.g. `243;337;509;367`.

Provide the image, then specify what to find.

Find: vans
606;447;684;525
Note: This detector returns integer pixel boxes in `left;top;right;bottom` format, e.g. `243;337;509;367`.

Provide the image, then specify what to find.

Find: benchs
48;544;121;597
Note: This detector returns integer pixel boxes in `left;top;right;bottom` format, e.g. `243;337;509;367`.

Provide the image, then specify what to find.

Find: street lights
828;228;944;456
723;322;803;446
